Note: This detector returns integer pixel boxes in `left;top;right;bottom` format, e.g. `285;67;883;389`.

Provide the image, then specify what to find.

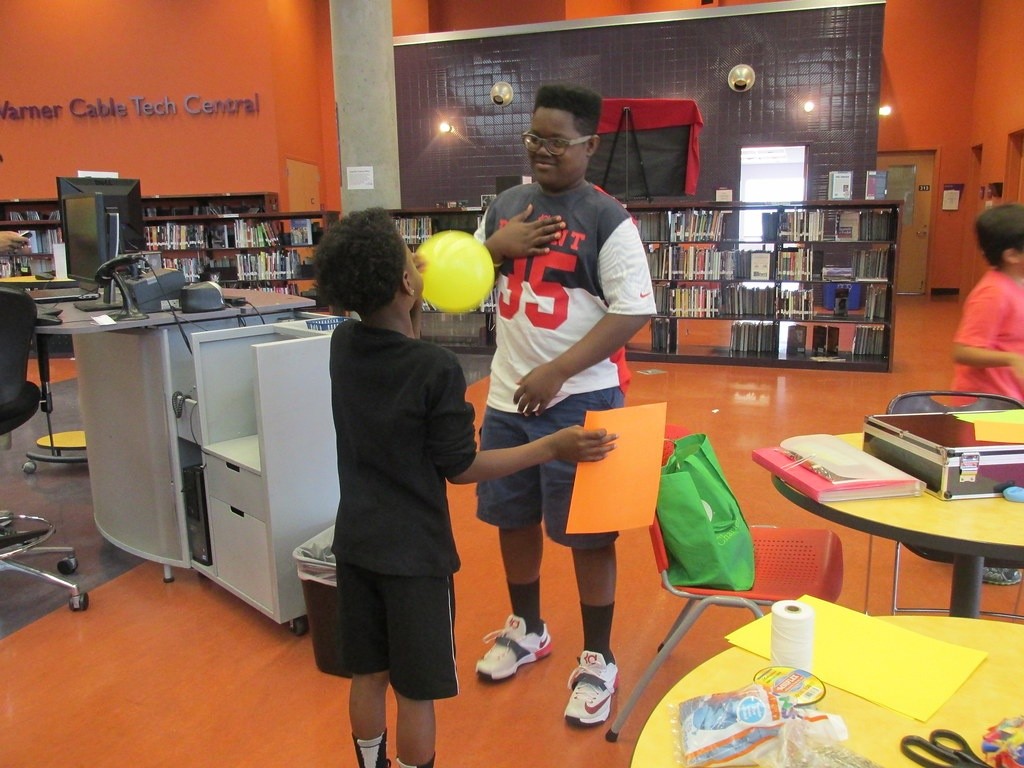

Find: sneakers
981;565;1021;585
565;650;620;728
476;615;554;681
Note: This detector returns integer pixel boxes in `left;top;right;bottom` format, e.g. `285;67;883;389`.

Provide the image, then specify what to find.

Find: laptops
28;282;101;304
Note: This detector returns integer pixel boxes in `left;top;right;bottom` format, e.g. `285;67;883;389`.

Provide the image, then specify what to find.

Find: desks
772;432;1024;620
0;274;81;288
628;614;1024;768
34;288;318;584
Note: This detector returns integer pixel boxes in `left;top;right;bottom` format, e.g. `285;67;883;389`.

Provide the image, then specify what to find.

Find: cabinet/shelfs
194;314;336;629
0;190;906;375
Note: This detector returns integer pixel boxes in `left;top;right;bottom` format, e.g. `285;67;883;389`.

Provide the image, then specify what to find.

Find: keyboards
37;306;63;316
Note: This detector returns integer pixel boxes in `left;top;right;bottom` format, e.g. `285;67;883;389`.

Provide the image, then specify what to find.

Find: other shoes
0;510;14;526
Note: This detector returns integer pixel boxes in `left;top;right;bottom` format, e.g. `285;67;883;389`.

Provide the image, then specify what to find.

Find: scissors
901;729;994;768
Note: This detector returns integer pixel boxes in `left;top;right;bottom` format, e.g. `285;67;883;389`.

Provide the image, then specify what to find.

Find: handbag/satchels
655;434;755;592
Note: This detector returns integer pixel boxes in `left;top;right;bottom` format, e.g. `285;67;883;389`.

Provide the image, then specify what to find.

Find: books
140;205;322;299
393;217;437;312
0;209;64;278
630;210;892;356
865;170;888;201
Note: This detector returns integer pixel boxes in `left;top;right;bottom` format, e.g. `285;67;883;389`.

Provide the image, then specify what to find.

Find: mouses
35;315;62;326
35;272;54;280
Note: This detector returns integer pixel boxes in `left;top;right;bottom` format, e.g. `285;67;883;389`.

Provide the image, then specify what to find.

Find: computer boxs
182;463;213;566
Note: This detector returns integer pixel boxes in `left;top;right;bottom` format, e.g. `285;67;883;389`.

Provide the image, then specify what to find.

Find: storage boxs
863;408;1024;503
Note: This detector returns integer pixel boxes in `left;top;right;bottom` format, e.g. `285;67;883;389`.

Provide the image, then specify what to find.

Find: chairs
863;389;1024;623
0;286;90;612
602;420;845;743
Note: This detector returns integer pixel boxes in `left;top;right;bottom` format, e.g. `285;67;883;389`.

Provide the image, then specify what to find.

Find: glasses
521;126;594;157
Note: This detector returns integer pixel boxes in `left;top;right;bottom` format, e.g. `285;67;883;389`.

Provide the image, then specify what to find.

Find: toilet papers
772;598;814;677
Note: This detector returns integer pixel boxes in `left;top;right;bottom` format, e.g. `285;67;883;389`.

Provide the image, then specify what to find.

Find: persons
949;204;1024;586
0;231;31;257
474;84;657;727
312;206;619;768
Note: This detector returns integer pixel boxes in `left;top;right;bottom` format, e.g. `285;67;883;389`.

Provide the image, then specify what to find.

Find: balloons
414;231;496;313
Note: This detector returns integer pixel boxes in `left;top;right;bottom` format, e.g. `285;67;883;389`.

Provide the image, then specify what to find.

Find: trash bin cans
292;523;353;678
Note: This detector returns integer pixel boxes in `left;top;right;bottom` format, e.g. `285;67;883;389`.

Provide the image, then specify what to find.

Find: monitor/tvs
57;177;147;312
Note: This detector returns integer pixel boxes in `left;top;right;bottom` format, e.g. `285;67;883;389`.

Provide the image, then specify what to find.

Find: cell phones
20;231;32;238
